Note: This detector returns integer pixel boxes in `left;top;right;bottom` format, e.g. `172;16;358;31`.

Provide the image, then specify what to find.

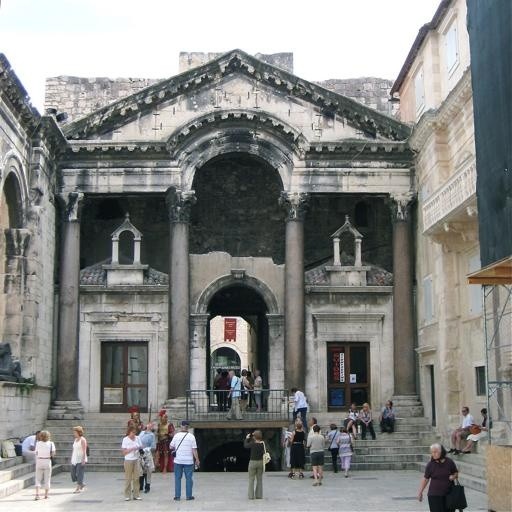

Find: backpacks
171;450;177;458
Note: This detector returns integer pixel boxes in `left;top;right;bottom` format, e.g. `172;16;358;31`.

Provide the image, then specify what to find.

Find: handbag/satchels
51;456;56;466
263;451;272;465
446;485;467;508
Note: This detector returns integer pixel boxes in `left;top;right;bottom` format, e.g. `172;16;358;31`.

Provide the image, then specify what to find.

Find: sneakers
289;472;323;487
448;448;472;455
353;434;376;440
186;496;195;500
174;496;182;501
73;485;86;493
125;483;151;502
35;495;51;500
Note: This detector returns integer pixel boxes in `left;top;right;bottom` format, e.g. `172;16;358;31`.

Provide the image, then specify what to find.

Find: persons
204;451;220;465
71;425;87;493
121;408;174;501
290;388;307;430
379;400;395;432
244;430;265;499
214;369;263;420
344;403;376;440
287;417;326;486
327;424;352;477
417;443;459;511
169;421;199;501
21;430;56;500
447;406;489;455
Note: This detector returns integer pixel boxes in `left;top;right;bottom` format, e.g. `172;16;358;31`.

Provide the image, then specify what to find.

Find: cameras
139;449;144;455
195;464;199;470
248;433;253;439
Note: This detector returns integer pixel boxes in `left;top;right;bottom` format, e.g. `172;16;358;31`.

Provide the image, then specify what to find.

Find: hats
181;420;189;427
